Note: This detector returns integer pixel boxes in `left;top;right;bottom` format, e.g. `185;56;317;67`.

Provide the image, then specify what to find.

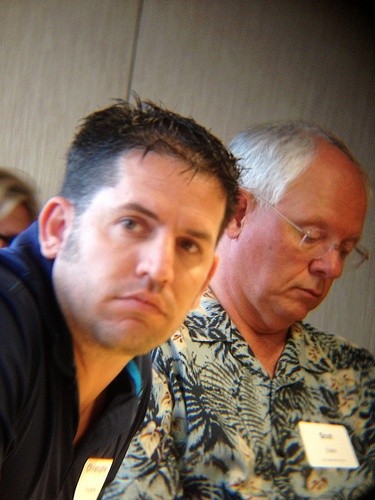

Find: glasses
0;232;18;245
252;193;370;271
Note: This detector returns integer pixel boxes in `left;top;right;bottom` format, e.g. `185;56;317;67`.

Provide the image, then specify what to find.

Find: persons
0;167;38;250
0;90;252;500
98;121;375;500
